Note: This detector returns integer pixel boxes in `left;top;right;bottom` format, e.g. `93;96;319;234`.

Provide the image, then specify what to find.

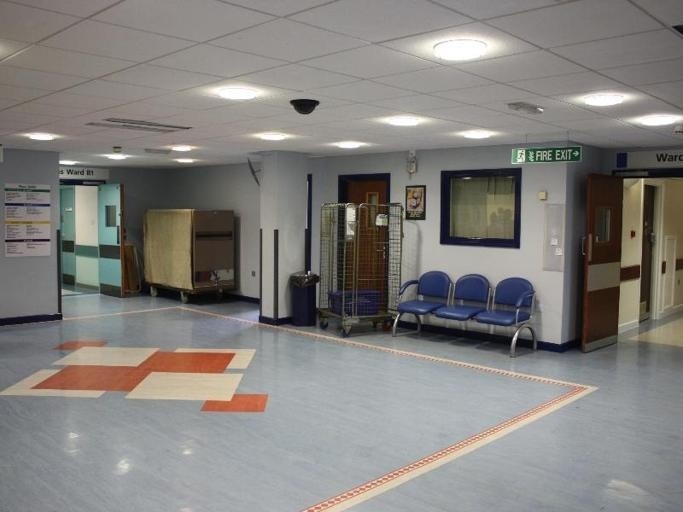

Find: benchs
393;270;537;357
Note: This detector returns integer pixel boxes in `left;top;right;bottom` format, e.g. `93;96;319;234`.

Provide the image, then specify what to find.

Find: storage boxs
329;289;380;316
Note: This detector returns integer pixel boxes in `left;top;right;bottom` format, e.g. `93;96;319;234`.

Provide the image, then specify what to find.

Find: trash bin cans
289;271;318;326
